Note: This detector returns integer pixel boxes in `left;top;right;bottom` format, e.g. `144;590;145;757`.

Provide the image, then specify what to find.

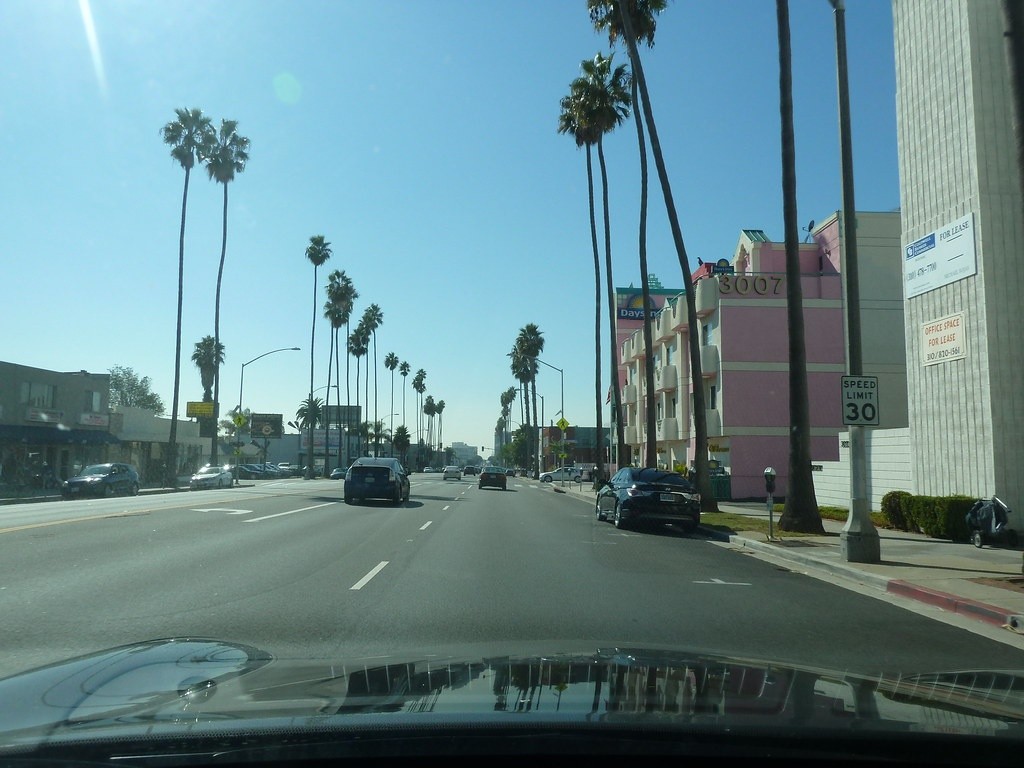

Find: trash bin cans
709;474;731;502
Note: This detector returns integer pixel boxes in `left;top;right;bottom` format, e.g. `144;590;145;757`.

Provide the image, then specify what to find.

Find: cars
443;465;461;480
342;456;411;505
464;464;516;477
60;461;141;498
595;466;702;534
423;466;432;473
478;466;507;491
230;461;325;479
188;463;234;491
538;466;582;483
329;467;346;479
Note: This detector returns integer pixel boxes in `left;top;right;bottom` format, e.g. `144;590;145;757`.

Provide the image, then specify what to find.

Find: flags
606;387;611;405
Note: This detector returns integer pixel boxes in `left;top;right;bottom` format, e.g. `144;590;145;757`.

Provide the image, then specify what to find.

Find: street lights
380;412;399;457
514;388;544;472
523;354;565;488
308;385;338;478
234;346;301;485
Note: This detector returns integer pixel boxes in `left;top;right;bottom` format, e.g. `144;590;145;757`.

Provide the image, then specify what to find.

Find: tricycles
965;495;1019;548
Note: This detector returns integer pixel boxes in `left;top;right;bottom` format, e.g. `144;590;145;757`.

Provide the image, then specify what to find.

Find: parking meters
763;466;776;541
